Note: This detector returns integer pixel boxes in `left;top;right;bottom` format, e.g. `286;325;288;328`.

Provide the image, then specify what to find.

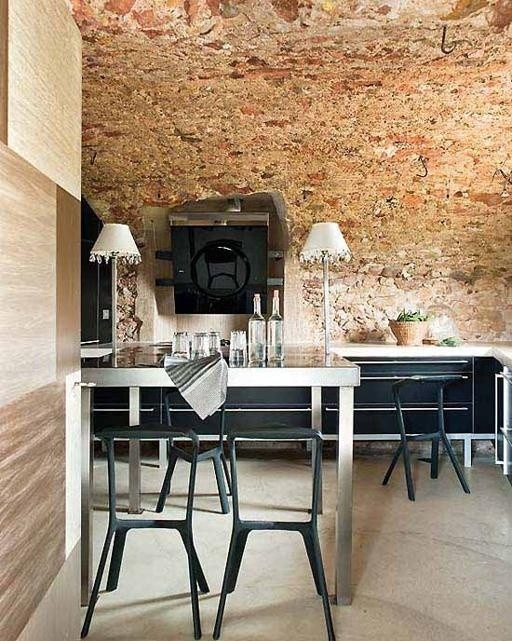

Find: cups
172;330;190;354
229;330;246;350
227;197;243;211
229;350;245;367
209;330;220;351
192;332;209;353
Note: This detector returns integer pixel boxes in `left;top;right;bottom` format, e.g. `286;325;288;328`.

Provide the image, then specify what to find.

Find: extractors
168;212;268;315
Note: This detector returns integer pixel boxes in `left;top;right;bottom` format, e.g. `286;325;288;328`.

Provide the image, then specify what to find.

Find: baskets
389;319;429;346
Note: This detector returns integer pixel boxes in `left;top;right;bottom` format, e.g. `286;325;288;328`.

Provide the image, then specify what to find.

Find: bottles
248;292;267;362
266;288;286;362
